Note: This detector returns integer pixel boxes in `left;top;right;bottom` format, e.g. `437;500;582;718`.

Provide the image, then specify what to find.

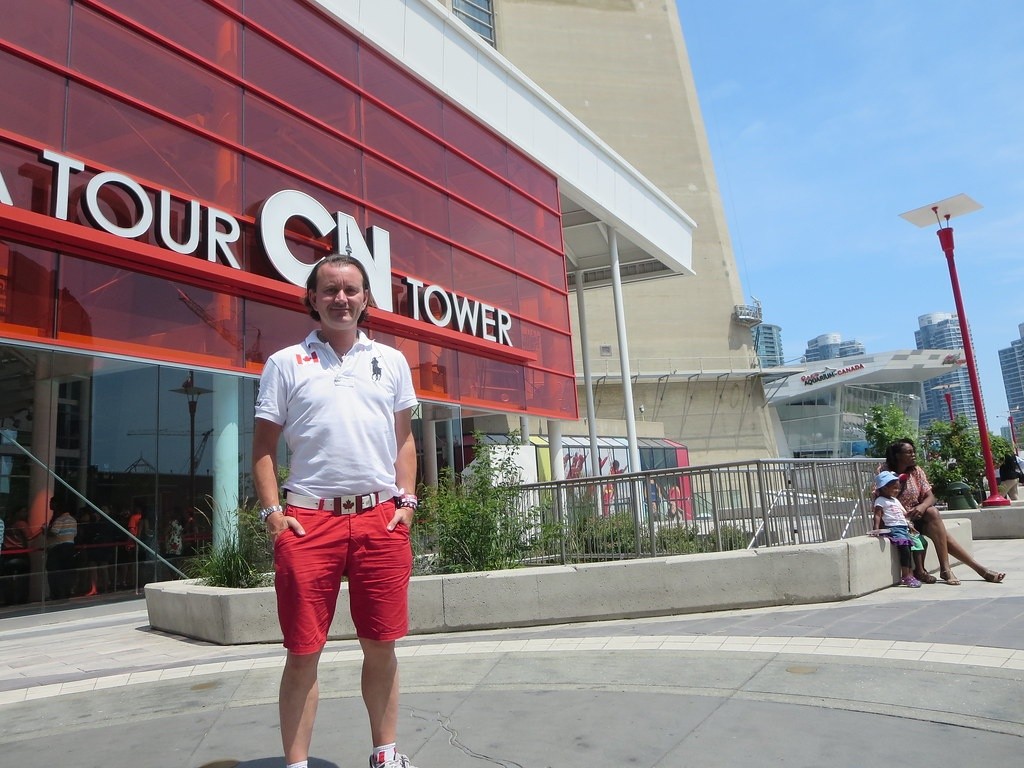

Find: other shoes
368;754;414;768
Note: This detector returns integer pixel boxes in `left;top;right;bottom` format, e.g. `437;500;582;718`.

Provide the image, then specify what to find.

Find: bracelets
399;494;418;511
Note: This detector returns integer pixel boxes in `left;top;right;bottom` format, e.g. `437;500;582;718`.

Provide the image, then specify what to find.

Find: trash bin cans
947;482;971;509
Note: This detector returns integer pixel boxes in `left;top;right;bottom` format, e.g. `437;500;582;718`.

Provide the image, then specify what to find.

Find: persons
869;471;937;588
926;434;940;460
873;438;1006;585
998;456;1024;500
0;496;199;597
668;482;681;518
252;254;419;768
564;453;629;516
644;480;661;514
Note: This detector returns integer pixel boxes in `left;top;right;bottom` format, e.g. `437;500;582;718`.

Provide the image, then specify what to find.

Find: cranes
124;430;254;517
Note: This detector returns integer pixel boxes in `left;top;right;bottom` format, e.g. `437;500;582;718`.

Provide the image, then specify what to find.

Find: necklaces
320;331;358;362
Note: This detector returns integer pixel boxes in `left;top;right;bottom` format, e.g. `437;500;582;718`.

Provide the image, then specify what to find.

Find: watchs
260;505;283;521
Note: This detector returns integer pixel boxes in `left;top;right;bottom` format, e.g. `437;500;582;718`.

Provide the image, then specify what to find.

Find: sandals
981;569;1006;582
916;572;938;583
939;570;961;585
901;577;923;588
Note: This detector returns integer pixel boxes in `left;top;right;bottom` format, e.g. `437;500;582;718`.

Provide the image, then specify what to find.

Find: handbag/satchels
1017;471;1024;484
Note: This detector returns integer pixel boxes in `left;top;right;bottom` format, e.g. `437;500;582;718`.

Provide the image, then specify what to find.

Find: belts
282;491;400;515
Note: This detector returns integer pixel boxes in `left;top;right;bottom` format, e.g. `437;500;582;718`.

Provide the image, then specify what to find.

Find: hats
873;471;899;489
890;471;907;484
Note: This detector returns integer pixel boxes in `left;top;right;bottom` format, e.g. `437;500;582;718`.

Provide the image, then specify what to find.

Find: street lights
930;382;960;421
897;192;1013;506
170;387;215;555
1003;409;1020;457
